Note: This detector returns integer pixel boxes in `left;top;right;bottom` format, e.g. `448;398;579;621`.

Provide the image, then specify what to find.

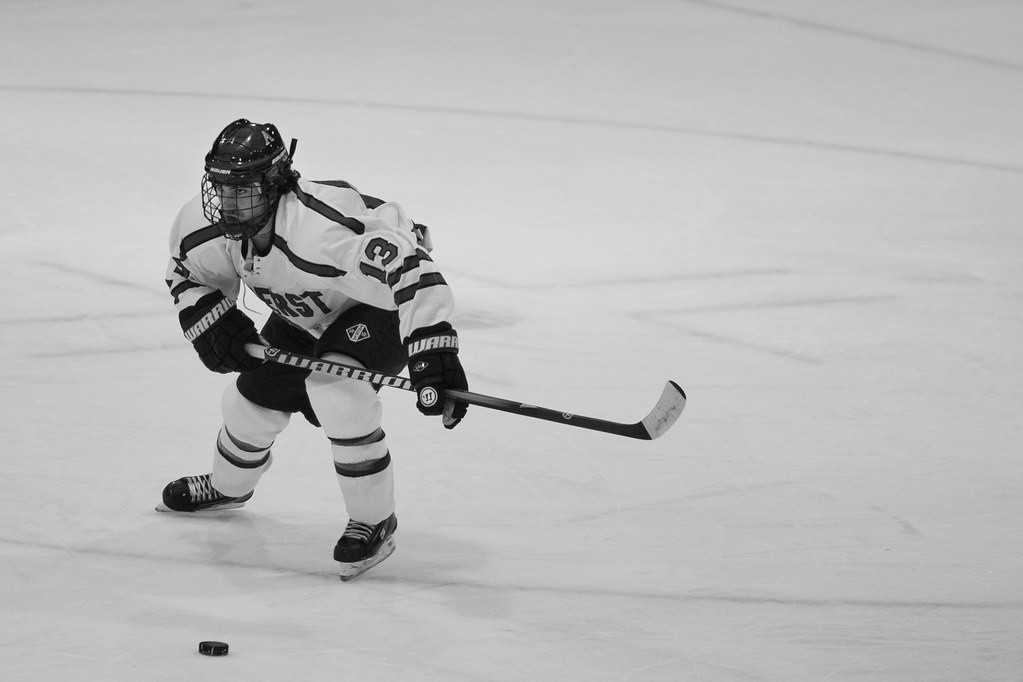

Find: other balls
199;641;229;657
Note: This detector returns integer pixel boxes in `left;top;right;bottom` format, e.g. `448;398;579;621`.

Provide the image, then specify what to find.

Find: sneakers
332;514;398;582
154;474;255;517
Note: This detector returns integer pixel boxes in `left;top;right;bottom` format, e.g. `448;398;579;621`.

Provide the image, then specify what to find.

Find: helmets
201;118;299;242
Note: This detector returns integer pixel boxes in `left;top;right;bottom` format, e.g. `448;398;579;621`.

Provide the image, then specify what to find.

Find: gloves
179;291;266;374
403;322;470;429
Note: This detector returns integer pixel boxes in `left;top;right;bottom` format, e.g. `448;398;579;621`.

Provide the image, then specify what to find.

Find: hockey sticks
244;343;689;441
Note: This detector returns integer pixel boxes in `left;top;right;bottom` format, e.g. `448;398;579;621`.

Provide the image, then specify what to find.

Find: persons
155;118;470;582
